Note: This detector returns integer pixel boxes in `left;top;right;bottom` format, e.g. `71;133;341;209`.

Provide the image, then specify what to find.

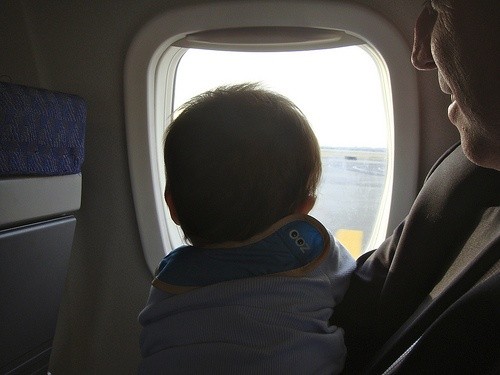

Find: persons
326;0;500;375
135;82;356;375
